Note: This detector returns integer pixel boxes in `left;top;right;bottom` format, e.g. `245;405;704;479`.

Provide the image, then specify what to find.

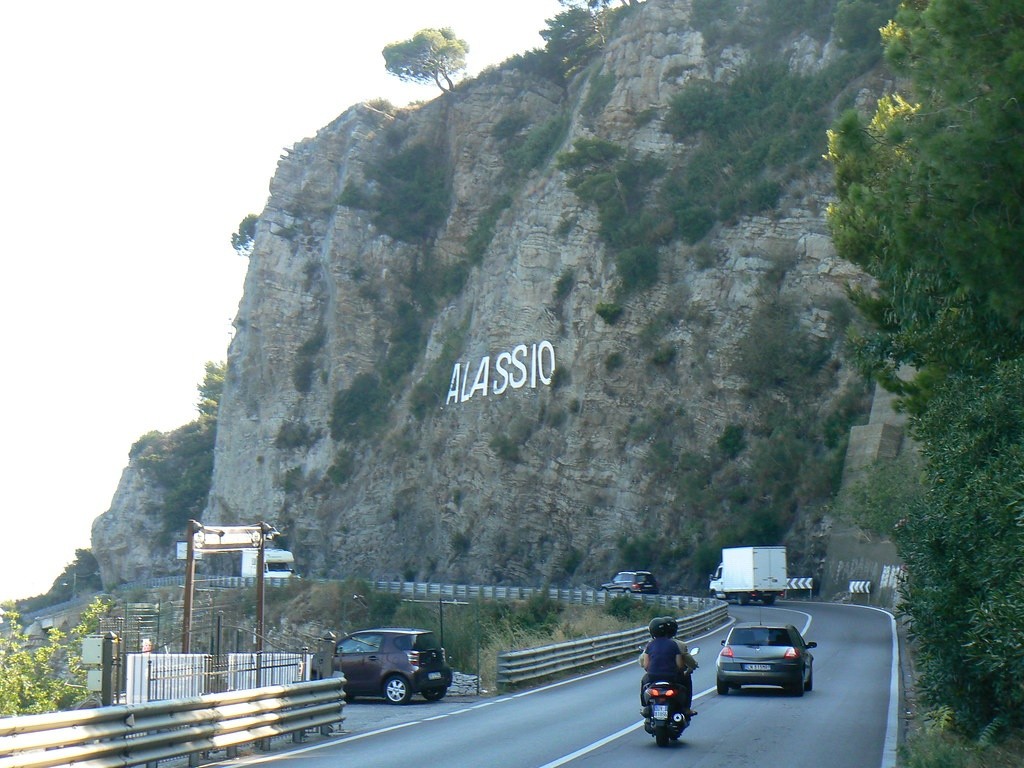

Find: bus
200;547;304;589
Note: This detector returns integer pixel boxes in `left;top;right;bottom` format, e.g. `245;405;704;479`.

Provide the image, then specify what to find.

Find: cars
302;626;454;707
714;623;817;698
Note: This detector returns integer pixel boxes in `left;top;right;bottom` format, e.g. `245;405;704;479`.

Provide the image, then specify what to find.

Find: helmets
662;616;678;636
648;617;670;638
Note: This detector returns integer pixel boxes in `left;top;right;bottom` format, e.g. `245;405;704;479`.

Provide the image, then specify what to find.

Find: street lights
178;518;225;653
256;519;280;651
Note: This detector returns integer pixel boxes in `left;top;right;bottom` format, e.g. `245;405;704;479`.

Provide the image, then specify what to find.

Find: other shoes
687;710;698;717
640;706;650;715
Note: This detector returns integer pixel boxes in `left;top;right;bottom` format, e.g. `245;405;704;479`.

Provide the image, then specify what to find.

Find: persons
637;618;698;716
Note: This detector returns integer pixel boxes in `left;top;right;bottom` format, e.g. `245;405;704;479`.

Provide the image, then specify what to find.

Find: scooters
637;646;701;747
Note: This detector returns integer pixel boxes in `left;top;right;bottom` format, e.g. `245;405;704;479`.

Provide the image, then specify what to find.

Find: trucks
708;546;788;607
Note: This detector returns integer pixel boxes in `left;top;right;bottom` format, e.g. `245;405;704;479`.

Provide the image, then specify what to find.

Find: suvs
600;571;661;594
